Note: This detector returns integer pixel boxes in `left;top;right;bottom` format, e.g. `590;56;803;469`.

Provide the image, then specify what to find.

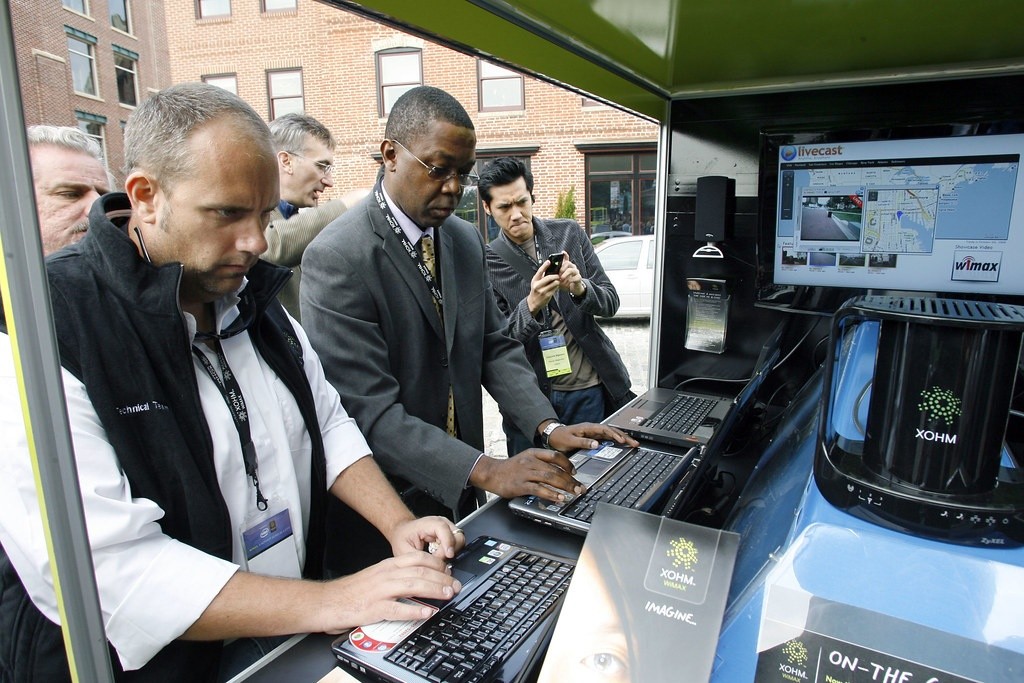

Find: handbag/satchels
605;383;638;417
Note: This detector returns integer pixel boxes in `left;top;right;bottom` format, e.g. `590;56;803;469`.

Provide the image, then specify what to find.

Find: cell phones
546;253;565;276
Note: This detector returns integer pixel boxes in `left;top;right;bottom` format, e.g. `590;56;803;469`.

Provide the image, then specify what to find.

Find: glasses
133;226;255;341
389;138;480;188
285;151;333;177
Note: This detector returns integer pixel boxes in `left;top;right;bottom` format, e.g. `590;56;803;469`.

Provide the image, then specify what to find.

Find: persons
298;86;640;581
257;113;374;324
0;81;466;683
26;124;116;257
477;155;631;459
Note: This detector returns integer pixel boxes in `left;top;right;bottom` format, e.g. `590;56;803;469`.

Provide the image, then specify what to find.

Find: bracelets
570;279;587;299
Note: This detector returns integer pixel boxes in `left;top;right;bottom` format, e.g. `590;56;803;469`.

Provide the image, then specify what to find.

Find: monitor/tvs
753;114;1024;315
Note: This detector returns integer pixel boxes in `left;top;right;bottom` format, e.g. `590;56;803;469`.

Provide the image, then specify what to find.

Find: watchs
541;422;567;450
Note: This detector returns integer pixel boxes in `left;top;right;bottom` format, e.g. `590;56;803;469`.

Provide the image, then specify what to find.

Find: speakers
695;175;728;242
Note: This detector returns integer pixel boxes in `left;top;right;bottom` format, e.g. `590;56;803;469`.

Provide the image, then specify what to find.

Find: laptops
598;314;792;451
330;446;699;683
508;348;781;538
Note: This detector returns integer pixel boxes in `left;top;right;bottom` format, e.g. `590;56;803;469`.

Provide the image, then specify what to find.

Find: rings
451;528;465;536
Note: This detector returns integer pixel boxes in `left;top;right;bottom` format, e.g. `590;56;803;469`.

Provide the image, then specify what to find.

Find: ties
420;236;458;440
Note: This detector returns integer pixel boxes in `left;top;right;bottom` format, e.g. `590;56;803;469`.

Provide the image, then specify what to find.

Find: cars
592;235;655;319
590;232;632;249
